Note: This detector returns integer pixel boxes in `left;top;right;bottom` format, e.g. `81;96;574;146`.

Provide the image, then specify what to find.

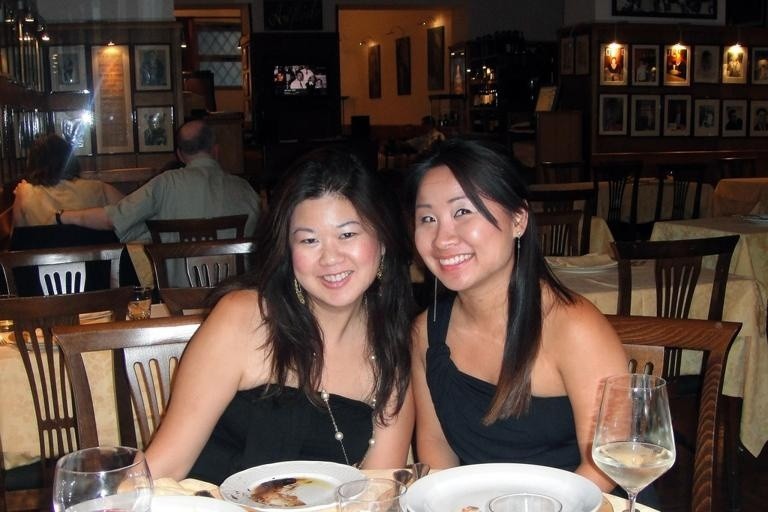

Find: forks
0;334;30;354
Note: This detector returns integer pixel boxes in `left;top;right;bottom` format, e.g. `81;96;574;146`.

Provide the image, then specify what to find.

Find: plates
550;252;618;273
6;325;61;349
65;492;247;512
403;461;603;512
218;460;368;511
79;309;114;324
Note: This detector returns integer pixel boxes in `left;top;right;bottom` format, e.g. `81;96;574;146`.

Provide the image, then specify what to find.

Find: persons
121;143;416;512
753;107;768;132
725;106;743;131
635;57;651;83
44;120;262;288
289;70;307;89
139;49;168;86
605;57;624;82
282;65;295;84
143;113;169;146
300;65;314;84
273;71;286;90
696;50;717;81
402;115;446;156
407;137;635;493
314;79;324;88
7;130;157;289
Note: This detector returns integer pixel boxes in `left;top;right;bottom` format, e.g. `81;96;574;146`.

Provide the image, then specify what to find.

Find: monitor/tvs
271;63;328;97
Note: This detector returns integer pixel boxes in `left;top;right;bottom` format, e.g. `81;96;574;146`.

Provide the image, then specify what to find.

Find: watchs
55;207;64;224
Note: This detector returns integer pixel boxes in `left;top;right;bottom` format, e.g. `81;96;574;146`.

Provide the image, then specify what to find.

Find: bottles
126;287;151;320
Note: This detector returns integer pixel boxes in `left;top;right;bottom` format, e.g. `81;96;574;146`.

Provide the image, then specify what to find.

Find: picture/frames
367;45;383;98
427;25;445;90
558;0;768;138
46;42;93;96
134;41;172;92
133;104;177;153
394;36;412;94
51;108;94;159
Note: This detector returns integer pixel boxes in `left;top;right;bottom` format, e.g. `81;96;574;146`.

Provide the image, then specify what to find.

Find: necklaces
301;296;369;467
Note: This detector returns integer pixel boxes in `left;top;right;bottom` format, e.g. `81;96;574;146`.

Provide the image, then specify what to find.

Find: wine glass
592;373;678;512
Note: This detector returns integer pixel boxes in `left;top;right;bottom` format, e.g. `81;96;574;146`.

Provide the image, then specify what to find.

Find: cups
339;478;408;511
52;446;154;512
484;493;561;512
